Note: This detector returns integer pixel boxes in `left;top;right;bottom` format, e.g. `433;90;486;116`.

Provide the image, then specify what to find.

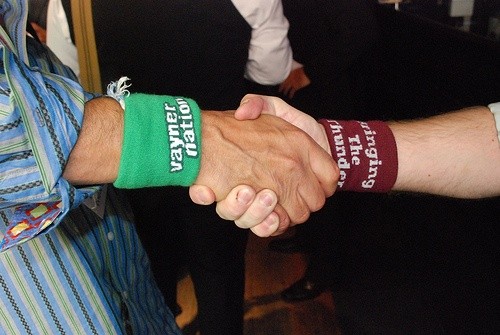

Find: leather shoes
280;260;343;301
270;232;318;256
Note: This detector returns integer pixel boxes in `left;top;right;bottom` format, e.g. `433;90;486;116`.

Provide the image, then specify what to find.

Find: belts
378;0;499;41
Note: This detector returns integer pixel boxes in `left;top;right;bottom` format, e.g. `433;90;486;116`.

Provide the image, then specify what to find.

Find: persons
276;1;379;302
190;93;499;237
62;0;293;333
0;0;341;335
